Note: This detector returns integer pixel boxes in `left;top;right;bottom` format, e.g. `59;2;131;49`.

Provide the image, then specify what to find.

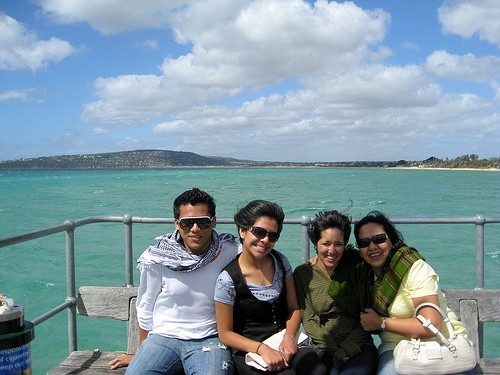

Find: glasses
248;226;279;243
355;234;388;248
177;216;213;230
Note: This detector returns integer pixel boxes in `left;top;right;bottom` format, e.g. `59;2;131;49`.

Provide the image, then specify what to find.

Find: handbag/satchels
392;302;476;375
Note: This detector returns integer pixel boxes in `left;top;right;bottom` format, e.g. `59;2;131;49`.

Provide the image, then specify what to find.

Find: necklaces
256;262;264;284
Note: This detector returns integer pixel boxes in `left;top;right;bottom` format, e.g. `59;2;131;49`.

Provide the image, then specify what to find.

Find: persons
305;211;485;375
214;200;329;375
293;210;378;375
108;188;243;375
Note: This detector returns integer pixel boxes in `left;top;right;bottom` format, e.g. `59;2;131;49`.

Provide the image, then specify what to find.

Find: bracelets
284;334;295;341
256;343;264;356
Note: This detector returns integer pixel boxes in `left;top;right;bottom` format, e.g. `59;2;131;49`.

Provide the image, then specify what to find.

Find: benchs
44;285;500;375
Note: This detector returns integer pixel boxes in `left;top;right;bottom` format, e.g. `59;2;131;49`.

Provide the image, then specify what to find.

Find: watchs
381;317;386;330
